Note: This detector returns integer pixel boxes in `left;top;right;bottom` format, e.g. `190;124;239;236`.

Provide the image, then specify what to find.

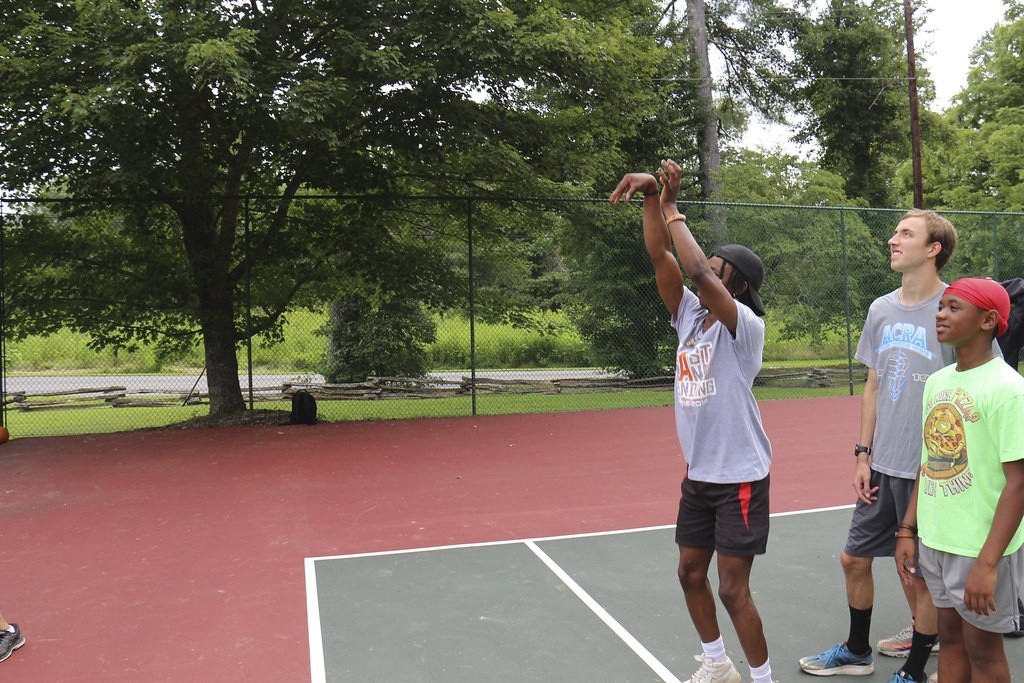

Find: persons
884;269;1023;683
606;156;784;681
872;332;1001;679
789;204;1004;683
997;586;1023;645
0;568;29;668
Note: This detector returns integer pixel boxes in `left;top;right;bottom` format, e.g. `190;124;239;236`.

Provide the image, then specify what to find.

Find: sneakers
930;671;938;681
798;643;875;676
682;656;741;683
1004;599;1024;637
875;627;940;657
886;670;927;682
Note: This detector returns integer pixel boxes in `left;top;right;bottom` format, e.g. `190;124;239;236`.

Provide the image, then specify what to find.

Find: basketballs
0;428;10;444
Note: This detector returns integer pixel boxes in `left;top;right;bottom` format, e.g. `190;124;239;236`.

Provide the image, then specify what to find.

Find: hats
711;245;767;316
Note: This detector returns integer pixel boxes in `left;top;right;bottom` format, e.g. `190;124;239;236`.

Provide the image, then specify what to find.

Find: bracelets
897;522;917;535
642;189;660;197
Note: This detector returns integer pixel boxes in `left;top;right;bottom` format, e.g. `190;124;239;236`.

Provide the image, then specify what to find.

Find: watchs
852;443;872;456
657;214;689;227
894;532;916;539
